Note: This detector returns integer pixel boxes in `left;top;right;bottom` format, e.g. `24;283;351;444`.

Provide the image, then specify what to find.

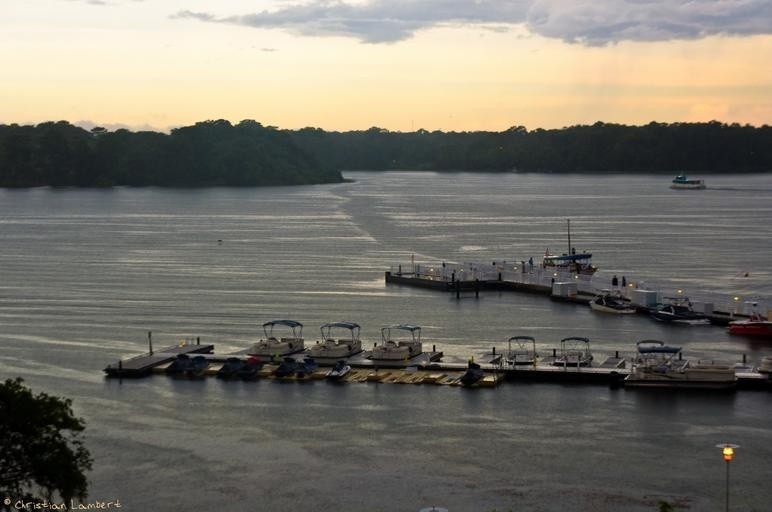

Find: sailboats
542;218;599;277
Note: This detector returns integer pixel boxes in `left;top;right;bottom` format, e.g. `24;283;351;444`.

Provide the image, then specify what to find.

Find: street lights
715;440;741;512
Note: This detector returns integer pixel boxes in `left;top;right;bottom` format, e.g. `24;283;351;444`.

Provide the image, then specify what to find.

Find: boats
636;337;669;371
589;288;636;315
505;332;538;367
256;315;424;362
553;335;592;366
650;295;707;322
728;299;772;336
670;169;704;190
624;346;738;392
